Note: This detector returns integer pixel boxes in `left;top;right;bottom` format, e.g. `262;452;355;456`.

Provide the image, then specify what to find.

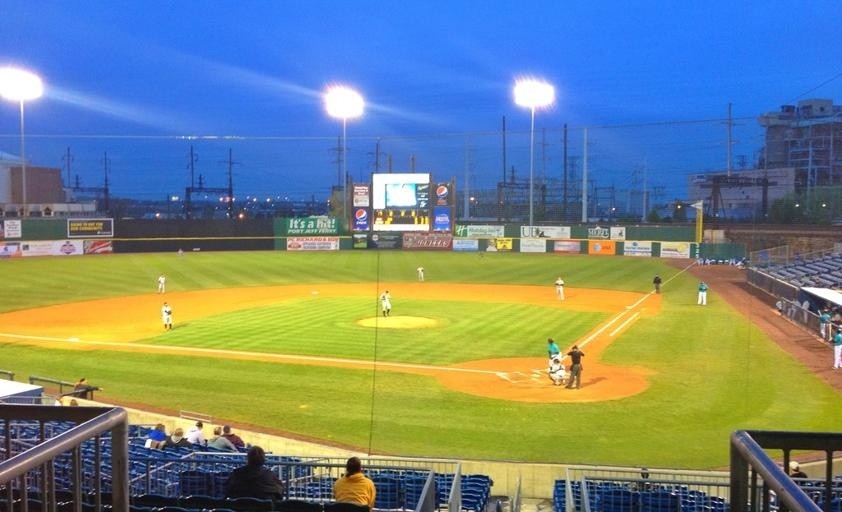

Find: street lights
1;67;45;221
325;88;365;221
511;81;556;225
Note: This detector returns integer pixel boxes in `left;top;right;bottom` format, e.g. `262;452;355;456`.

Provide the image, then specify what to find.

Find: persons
555;277;564;301
162;302;172;330
790;461;808;486
158;274;165;294
816;277;820;286
547;339;562;366
177;248;184;256
697;282;708;305
565;345;584;389
735;257;747;267
222;445;285;504
73;378;103;399
547;359;566;386
632;468;656;492
145;420;245;453
416;265;424;281
332;457;376;512
379;291;391;317
776;296;842;369
653;274;661;294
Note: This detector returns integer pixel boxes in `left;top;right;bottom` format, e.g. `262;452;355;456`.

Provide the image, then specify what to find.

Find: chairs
553;471;841;511
0;420;493;512
758;245;842;294
